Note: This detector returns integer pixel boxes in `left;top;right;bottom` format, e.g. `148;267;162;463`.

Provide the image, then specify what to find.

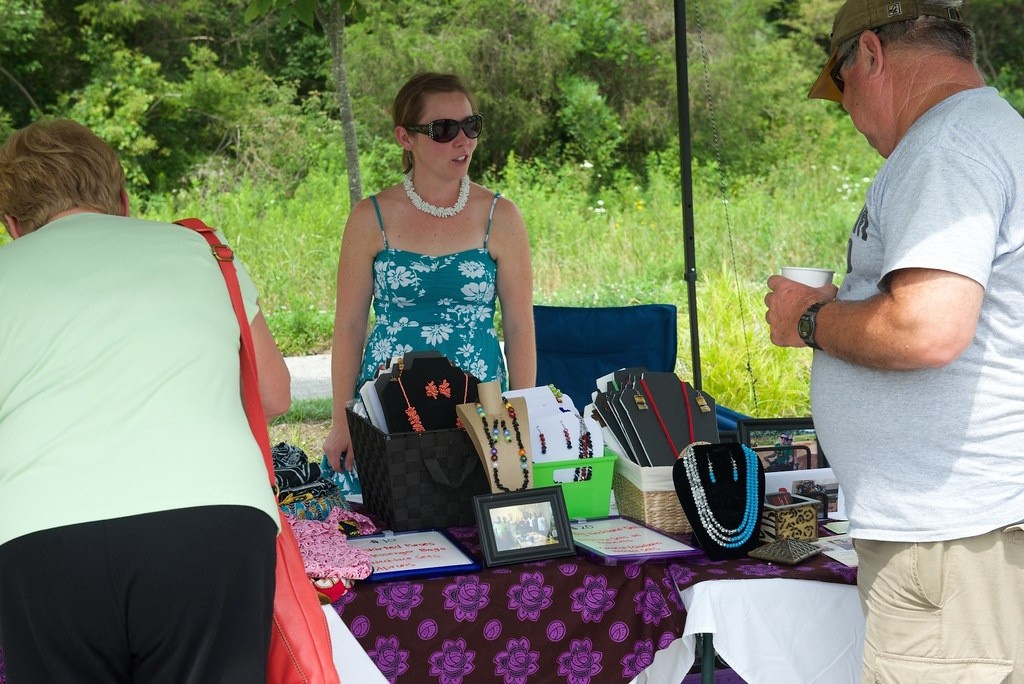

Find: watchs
798;302;824;352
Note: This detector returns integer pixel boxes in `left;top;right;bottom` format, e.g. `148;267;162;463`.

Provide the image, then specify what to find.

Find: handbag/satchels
265;507;342;684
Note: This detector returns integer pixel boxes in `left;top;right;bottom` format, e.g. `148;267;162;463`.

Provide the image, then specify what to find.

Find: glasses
404;115;483;143
829;27;883;93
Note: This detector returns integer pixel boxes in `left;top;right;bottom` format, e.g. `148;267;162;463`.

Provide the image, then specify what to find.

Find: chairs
532;303;754;433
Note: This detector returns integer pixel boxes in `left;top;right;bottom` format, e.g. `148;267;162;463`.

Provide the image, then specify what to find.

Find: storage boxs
611;452;693;534
344;405;487;536
758;490;821;543
532;447;621;517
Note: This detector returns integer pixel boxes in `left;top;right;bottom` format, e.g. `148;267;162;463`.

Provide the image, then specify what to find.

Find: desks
323;504;869;684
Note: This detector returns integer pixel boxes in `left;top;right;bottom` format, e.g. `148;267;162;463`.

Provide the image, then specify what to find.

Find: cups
780;267;835;288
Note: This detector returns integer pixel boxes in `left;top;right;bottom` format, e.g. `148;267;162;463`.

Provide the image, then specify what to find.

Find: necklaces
404;168;470;218
683;442;758;548
398;364;468;432
574;412;593;484
641;379;694;460
475;396;529;492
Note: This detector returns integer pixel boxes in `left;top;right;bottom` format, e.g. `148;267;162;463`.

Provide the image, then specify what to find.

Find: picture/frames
736;419;828;473
470;485;575;566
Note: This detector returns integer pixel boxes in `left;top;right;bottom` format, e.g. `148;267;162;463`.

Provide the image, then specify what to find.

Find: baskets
607;447;695;534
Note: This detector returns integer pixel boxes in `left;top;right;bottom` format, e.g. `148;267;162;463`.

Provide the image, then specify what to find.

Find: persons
0;121;291;684
493;512;547;552
764;433;798;471
320;73;537;496
764;0;1024;684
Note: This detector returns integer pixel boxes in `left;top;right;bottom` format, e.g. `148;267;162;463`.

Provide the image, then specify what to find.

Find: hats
807;0;967;102
